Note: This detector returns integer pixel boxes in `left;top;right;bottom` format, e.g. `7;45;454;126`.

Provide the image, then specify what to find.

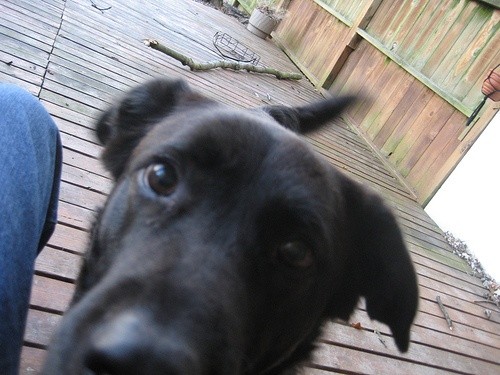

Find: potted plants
247;0;290;39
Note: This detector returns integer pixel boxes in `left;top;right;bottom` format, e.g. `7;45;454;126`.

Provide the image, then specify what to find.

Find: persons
0;82;62;375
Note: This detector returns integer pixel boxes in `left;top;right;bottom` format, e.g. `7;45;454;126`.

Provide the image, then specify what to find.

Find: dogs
38;74;421;375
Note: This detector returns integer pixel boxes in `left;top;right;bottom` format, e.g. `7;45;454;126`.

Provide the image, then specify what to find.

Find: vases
482;69;500;102
